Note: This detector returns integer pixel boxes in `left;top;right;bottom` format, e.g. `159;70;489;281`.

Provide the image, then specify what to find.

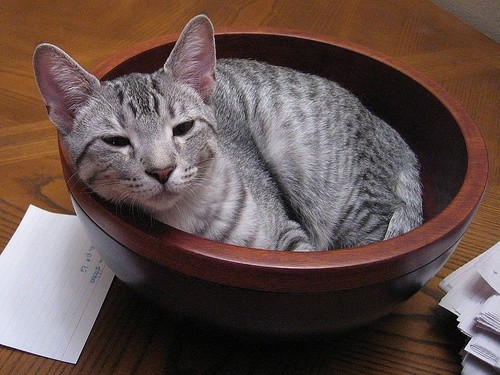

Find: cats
32;13;423;253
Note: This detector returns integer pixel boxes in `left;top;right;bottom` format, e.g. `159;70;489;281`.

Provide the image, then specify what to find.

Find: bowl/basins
57;24;489;332
